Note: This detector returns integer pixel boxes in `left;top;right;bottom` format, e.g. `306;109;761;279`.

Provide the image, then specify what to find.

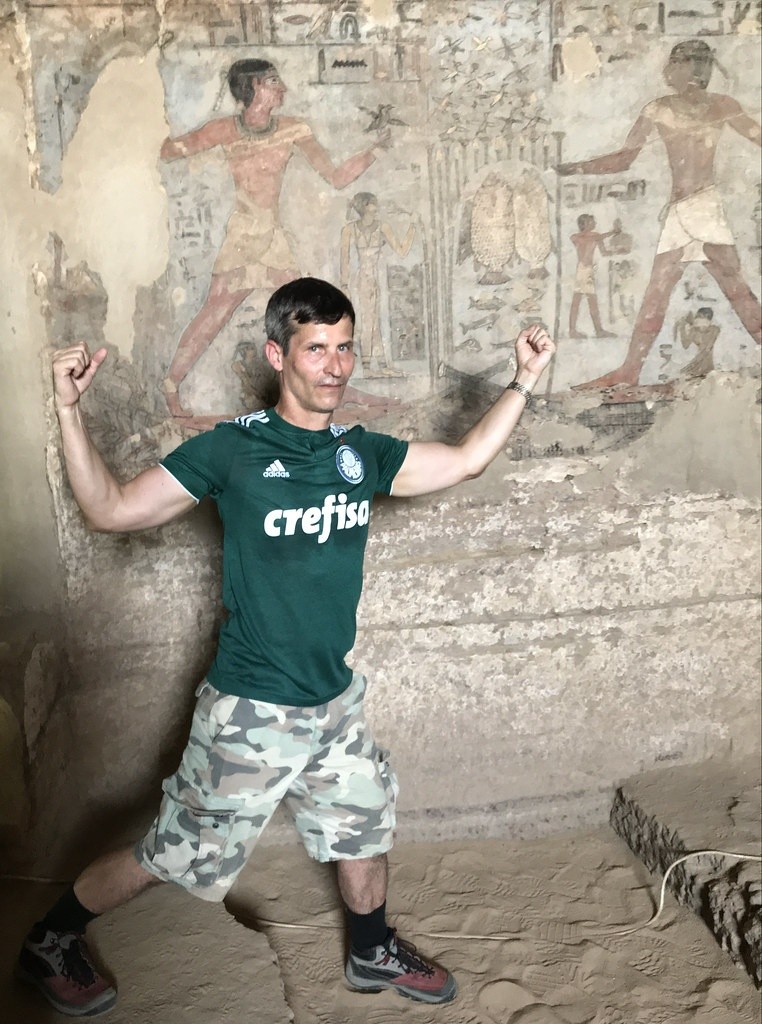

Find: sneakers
13;922;119;1019
341;926;458;1004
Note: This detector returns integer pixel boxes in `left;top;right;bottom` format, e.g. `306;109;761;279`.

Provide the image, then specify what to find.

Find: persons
13;277;556;1019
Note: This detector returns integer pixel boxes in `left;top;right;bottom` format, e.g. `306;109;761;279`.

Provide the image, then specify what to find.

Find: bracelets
506;381;532;409
